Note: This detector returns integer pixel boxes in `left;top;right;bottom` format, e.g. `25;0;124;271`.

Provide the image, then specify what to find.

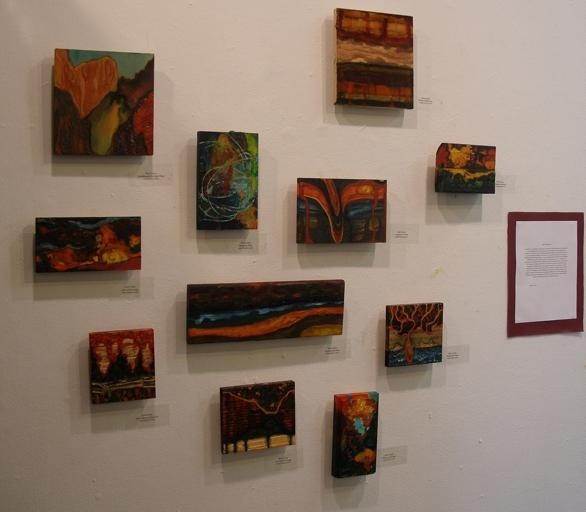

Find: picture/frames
506;211;584;337
